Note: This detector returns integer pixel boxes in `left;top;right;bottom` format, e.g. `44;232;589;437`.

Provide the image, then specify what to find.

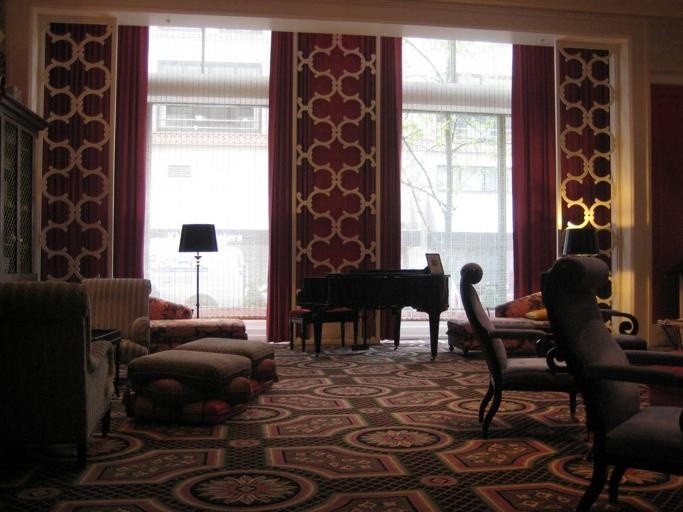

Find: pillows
522;304;548;322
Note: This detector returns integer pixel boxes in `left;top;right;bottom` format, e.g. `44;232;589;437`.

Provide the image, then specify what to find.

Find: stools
174;334;283;399
118;348;254;429
287;308;360;352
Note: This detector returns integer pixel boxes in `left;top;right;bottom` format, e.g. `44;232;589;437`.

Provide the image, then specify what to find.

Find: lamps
559;226;605;257
178;221;219;320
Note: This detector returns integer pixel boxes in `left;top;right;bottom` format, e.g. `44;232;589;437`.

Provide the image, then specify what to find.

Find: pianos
297;268;450;357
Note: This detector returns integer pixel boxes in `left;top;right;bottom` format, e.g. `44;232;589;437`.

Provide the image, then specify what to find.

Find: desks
88;327;128;402
654;317;683;350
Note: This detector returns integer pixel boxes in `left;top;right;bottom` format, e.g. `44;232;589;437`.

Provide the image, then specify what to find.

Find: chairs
146;316;247;352
0;278;121;474
536;255;683;510
78;276;153;366
446;290;557;360
577;253;647;353
460;263;582;430
